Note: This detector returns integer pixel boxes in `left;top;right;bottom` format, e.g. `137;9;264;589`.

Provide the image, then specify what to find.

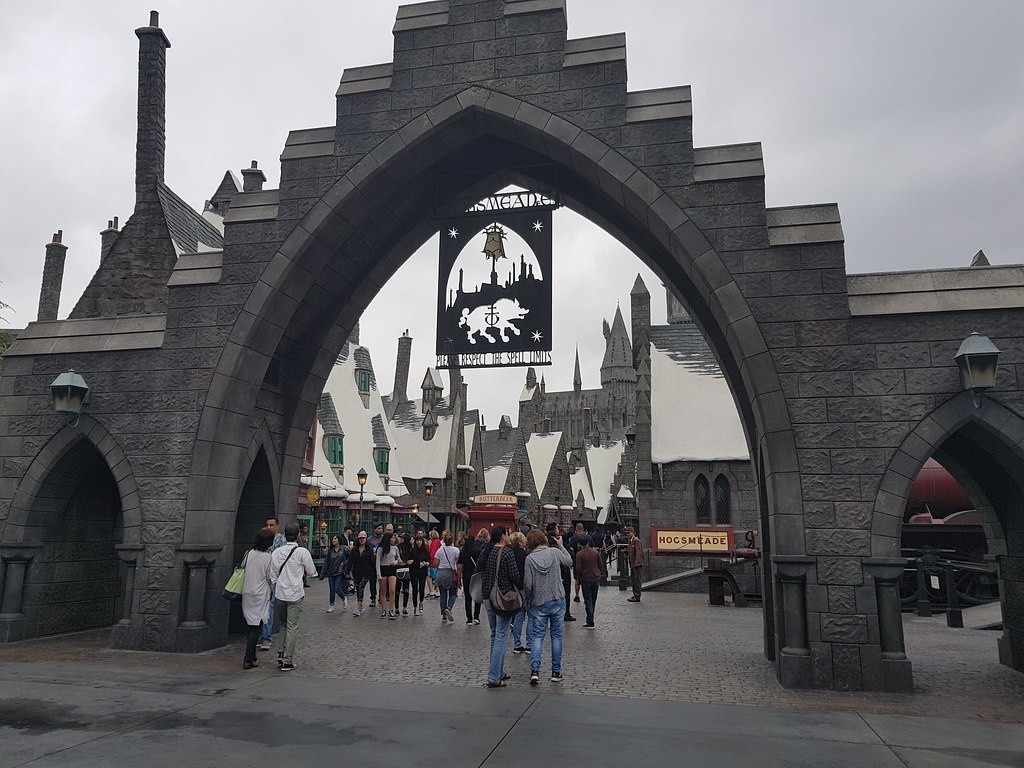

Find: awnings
416;512;441;523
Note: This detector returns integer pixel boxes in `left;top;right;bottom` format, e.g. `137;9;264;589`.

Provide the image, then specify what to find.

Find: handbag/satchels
469;571;483;604
489;583;524;617
453;572;458;585
221;566;244;602
396;565;411;581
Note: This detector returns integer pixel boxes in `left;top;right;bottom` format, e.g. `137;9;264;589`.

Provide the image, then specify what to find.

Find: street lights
357;468;369;533
425;480;434;531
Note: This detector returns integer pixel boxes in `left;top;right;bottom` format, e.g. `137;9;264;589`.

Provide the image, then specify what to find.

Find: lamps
955;330;1002;409
50;369;90;428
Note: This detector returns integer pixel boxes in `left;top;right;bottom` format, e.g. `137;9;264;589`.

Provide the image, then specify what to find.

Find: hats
284;522;301;535
398;533;411;541
358;530;367;538
624;527;634;533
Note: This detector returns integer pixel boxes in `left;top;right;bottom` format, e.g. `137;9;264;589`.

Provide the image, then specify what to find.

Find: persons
375;533;403;619
458;535;485;625
339;523;476;604
410;538;431;616
624;527;646;602
433;532;459;621
342;531;373;616
297;525;311;588
395;534;414;617
547;522;577;623
319;534;352;612
476;524;621;567
575;533;601;629
570;523;595;602
473;525;523;688
256;517;287;649
508;532;544;655
270;522;318;671
222;530;274;669
523;529;573;685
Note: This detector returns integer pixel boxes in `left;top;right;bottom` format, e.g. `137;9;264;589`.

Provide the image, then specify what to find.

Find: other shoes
257;639;270;650
531;672;540;684
304;583;311;587
514;646;531;654
550;672;564;682
628;596;639;601
582;623;596;629
244;658;259;668
488;680;506;686
574;596;580;601
325;600;480;625
428;590;440;597
503;675;511;680
277;653;297;670
563;616;577;621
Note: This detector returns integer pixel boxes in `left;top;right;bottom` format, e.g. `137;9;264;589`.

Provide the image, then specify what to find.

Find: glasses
377;527;383;530
